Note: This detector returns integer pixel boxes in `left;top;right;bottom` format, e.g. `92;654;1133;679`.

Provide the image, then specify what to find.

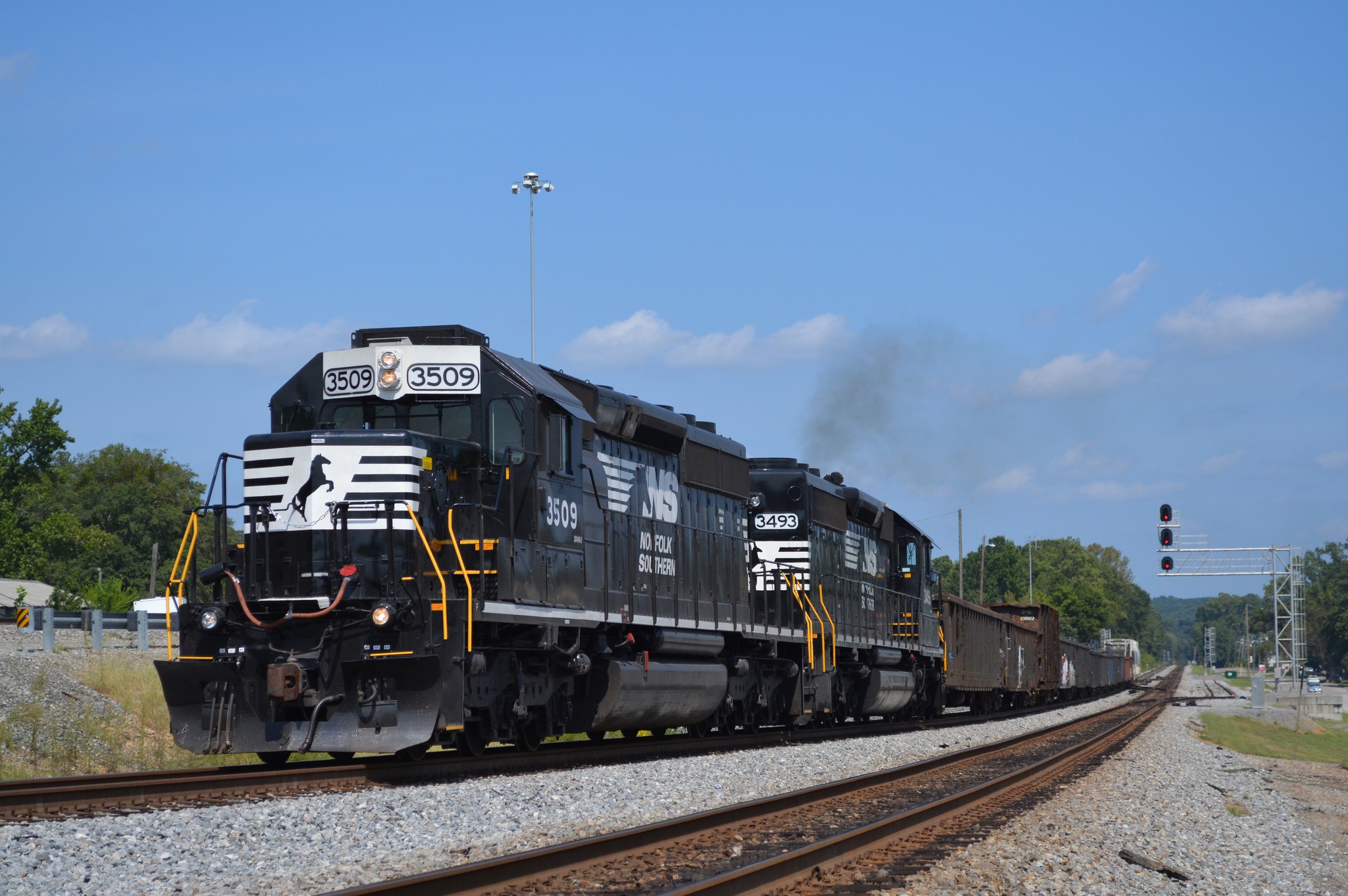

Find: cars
1307;682;1323;694
1277;663;1341;684
1307;675;1320;684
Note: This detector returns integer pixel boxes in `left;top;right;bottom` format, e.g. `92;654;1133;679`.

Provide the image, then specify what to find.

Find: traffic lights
1162;556;1173;570
1160;504;1172;522
1161;529;1173;546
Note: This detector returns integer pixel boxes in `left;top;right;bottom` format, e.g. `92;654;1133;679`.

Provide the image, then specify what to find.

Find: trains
152;323;1137;766
